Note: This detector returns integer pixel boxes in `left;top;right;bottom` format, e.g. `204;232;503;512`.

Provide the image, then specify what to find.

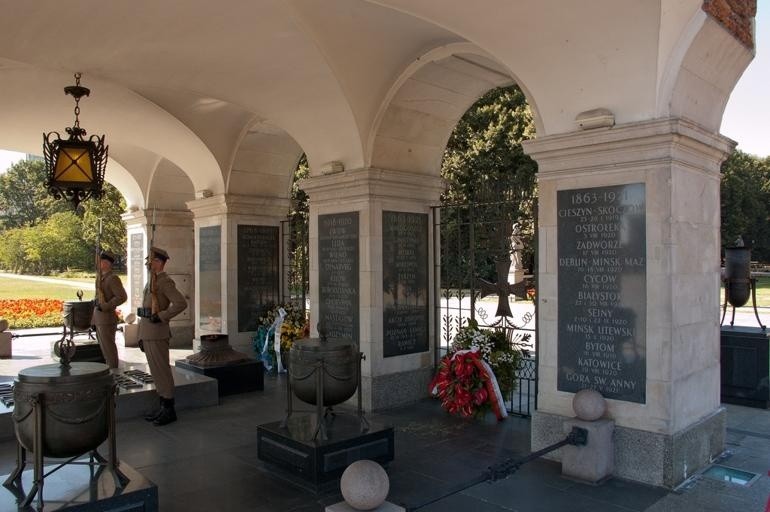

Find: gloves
139;340;144;352
91;325;96;331
96;304;102;312
150;313;161;323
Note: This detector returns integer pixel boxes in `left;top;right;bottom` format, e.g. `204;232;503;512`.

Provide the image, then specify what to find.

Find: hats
145;247;169;259
99;249;114;263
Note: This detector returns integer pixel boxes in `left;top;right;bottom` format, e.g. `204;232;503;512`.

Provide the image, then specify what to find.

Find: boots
144;396;176;425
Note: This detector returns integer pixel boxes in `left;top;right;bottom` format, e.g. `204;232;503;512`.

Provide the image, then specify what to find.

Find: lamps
43;71;112;211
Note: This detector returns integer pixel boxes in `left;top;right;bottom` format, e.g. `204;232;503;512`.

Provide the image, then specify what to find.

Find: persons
136;246;187;426
91;253;127;369
